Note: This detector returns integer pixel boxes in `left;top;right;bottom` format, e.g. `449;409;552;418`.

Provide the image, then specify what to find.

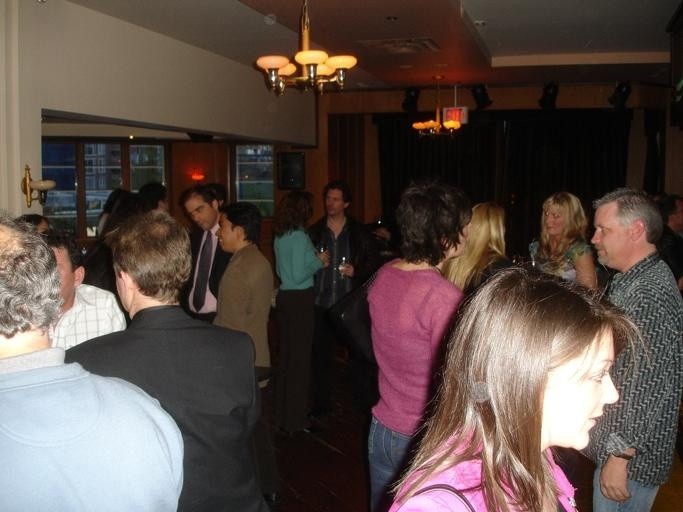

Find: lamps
19;164;56;208
256;1;358;99
411;76;462;139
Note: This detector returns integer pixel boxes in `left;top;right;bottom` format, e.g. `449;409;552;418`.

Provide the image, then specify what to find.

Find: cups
316;244;328;254
337;256;350;279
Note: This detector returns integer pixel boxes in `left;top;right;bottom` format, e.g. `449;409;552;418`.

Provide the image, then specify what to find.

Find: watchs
610;449;634;461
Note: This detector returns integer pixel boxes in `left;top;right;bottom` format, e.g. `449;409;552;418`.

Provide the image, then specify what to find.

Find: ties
193;230;213;313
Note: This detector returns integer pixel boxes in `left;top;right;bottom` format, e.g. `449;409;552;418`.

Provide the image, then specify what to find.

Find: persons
576;189;681;511
9;180;681;422
361;180;468;512
0;210;186;511
386;266;643;511
62;209;268;512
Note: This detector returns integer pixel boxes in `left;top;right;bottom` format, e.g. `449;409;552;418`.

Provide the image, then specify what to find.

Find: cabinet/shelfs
234;145;273;204
